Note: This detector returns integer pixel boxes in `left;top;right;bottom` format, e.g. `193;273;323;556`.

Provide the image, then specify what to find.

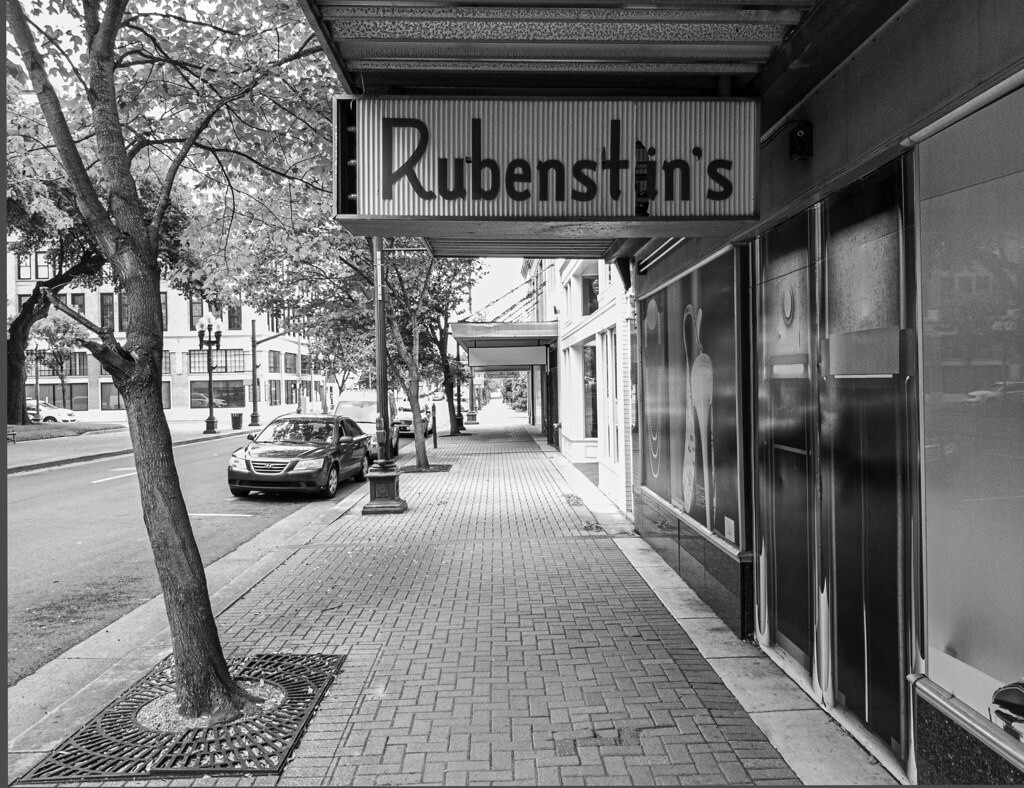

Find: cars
393;401;432;436
432;392;446;401
25;397;77;424
28;409;42;423
228;411;373;499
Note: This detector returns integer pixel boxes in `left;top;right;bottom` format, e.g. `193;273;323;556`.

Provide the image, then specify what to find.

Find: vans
330;389;399;462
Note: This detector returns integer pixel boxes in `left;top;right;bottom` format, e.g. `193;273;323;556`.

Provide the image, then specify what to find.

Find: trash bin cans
231;413;243;430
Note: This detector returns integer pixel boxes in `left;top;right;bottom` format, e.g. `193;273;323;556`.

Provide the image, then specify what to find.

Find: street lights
195;311;224;434
